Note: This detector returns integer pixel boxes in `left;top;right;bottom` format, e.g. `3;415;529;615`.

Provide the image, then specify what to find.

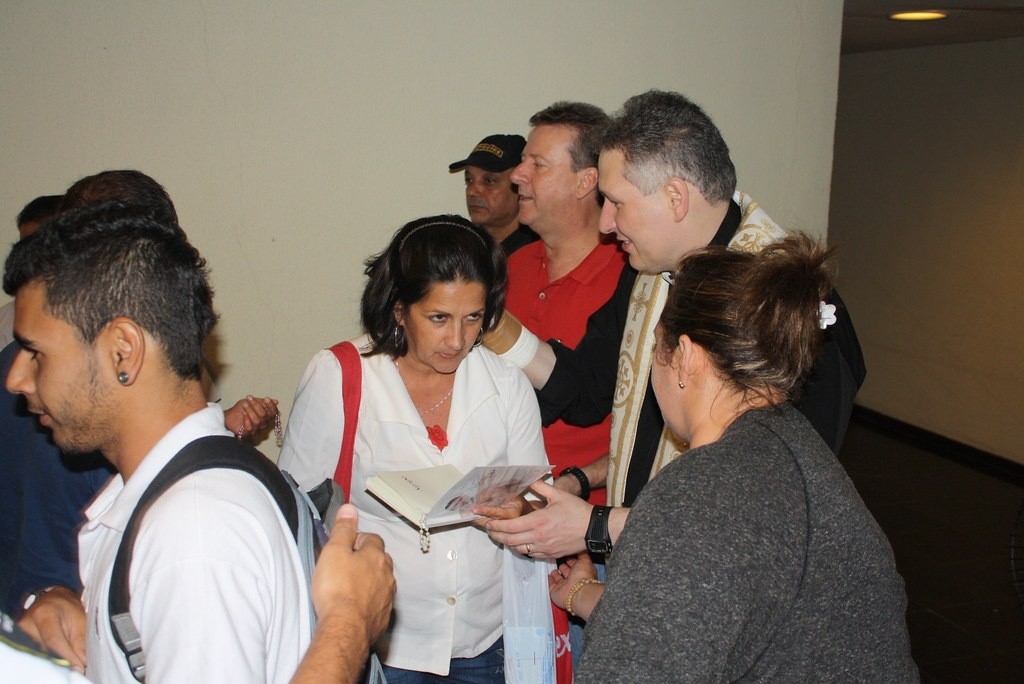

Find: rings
526;544;531;554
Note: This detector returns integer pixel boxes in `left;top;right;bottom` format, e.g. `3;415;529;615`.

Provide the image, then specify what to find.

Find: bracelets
21;586;57;614
566;578;604;615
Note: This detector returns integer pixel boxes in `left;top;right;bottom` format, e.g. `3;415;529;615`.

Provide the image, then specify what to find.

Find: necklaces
238;406;281;439
420;514;430;546
396;362;453;414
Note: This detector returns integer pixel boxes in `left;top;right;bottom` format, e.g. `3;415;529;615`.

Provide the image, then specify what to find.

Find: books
364;464;556;529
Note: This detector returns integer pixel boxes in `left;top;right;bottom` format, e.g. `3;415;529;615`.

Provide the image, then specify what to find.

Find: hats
448;134;527;173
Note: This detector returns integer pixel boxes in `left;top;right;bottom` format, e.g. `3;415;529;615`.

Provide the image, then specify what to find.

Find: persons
504;100;629;684
278;214;554;684
449;134;544;255
445;479;530;513
547;233;921;684
477;89;865;569
0;170;396;684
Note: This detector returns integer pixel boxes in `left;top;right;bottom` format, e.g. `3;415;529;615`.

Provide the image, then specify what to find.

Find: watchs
585;505;613;564
561;466;590;500
